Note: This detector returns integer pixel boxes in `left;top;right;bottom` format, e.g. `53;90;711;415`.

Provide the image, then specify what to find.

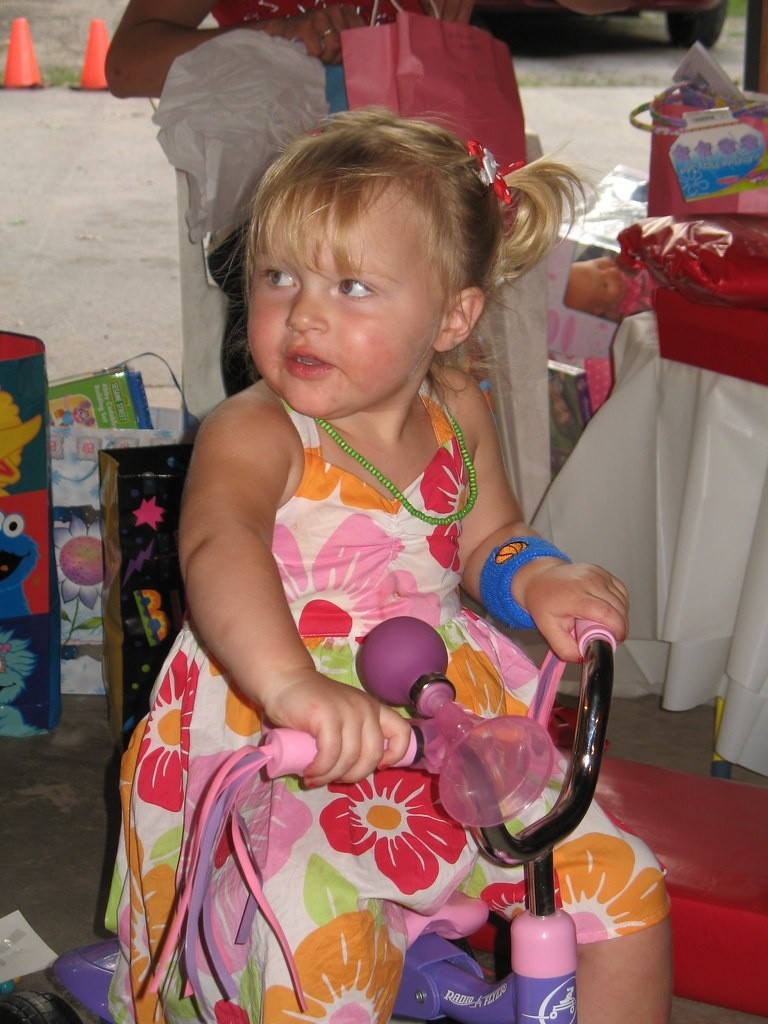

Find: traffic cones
68;18;113;93
2;15;46;88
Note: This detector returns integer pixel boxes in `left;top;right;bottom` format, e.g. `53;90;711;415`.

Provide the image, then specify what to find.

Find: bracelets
479;536;572;629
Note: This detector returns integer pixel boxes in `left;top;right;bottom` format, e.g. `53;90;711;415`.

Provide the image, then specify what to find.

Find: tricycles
0;613;619;1024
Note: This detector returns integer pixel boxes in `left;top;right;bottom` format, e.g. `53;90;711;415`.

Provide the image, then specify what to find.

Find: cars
466;0;733;52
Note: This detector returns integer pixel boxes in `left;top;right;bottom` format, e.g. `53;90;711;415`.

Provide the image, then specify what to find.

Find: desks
524;311;767;790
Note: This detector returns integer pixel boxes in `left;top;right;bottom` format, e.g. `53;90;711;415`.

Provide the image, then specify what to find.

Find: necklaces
316;406;478;525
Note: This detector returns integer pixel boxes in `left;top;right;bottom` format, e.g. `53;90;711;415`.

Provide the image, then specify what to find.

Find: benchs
594;753;768;1019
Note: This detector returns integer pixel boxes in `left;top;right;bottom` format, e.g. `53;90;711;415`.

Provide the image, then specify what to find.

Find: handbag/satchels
341;0;527;162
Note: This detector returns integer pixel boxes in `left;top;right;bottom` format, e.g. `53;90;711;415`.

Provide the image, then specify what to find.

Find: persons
100;107;672;1022
105;0;473;99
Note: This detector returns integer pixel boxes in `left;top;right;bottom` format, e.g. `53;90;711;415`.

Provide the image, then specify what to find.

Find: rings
322;28;334;36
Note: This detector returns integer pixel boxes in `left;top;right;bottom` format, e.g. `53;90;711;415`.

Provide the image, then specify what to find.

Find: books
672;40;744;101
